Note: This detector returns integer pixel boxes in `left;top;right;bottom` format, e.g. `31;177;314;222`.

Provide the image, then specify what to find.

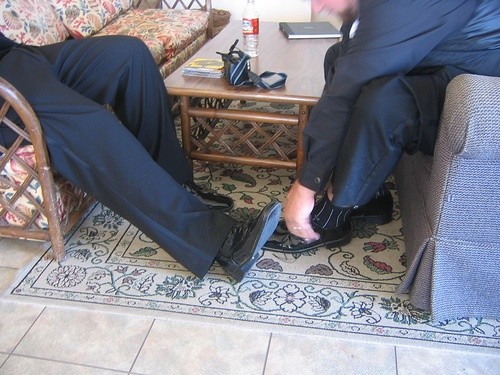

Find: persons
261;0;500;253
0;29;282;283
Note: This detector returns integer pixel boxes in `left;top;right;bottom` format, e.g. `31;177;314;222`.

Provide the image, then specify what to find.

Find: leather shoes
180;180;236;210
261;218;352;253
350;187;393;225
215;201;281;284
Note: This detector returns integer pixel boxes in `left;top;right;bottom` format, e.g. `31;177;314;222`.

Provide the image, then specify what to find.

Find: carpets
2;101;499;350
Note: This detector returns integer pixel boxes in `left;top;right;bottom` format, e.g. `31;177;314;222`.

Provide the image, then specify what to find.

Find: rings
293;226;301;230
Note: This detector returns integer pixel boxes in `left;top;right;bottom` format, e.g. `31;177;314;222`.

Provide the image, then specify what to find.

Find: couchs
398;74;500;322
1;0;232;265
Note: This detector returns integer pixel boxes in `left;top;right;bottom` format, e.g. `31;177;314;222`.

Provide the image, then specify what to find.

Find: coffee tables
159;21;342;176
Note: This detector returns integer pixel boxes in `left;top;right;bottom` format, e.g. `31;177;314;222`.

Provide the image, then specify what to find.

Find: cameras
221;40;248;85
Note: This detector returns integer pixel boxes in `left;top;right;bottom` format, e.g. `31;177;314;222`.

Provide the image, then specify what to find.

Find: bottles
243;0;260;57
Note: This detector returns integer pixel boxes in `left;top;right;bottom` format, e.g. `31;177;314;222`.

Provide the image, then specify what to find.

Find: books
279;21;342;40
